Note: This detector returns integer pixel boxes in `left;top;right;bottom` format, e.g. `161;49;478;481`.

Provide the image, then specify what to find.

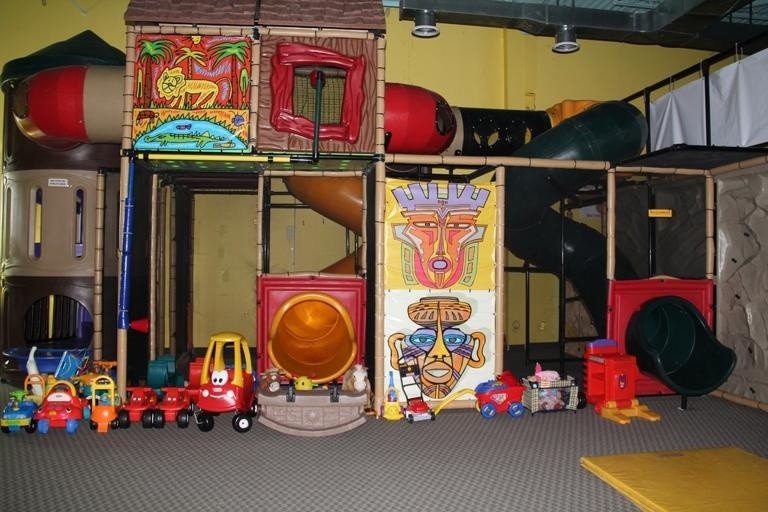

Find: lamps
412;8;439;38
549;24;580;54
